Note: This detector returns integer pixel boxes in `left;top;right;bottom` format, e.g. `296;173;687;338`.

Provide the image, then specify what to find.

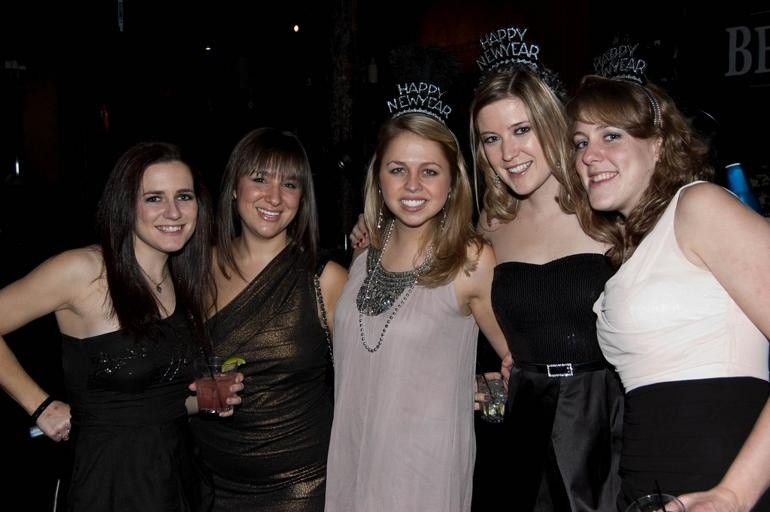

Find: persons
0;136;246;512
198;119;351;511
324;113;509;510
348;67;630;511
499;77;769;512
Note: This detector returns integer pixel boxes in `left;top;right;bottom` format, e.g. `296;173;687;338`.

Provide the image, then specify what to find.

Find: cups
624;494;687;512
477;378;508;424
194;355;237;415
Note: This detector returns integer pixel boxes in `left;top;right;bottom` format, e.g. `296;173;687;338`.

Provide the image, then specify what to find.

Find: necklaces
355;215;440;354
137;260;171;294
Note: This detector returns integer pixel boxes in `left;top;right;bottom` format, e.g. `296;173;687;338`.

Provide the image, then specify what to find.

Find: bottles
725;161;761;214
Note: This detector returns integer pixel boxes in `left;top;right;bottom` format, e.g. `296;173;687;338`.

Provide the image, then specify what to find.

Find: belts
512;362;606;377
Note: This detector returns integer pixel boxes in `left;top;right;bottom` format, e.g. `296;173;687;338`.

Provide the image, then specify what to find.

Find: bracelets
29;394;56;423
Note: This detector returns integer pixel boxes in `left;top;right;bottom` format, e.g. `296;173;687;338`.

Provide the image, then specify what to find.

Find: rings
60;429;70;442
